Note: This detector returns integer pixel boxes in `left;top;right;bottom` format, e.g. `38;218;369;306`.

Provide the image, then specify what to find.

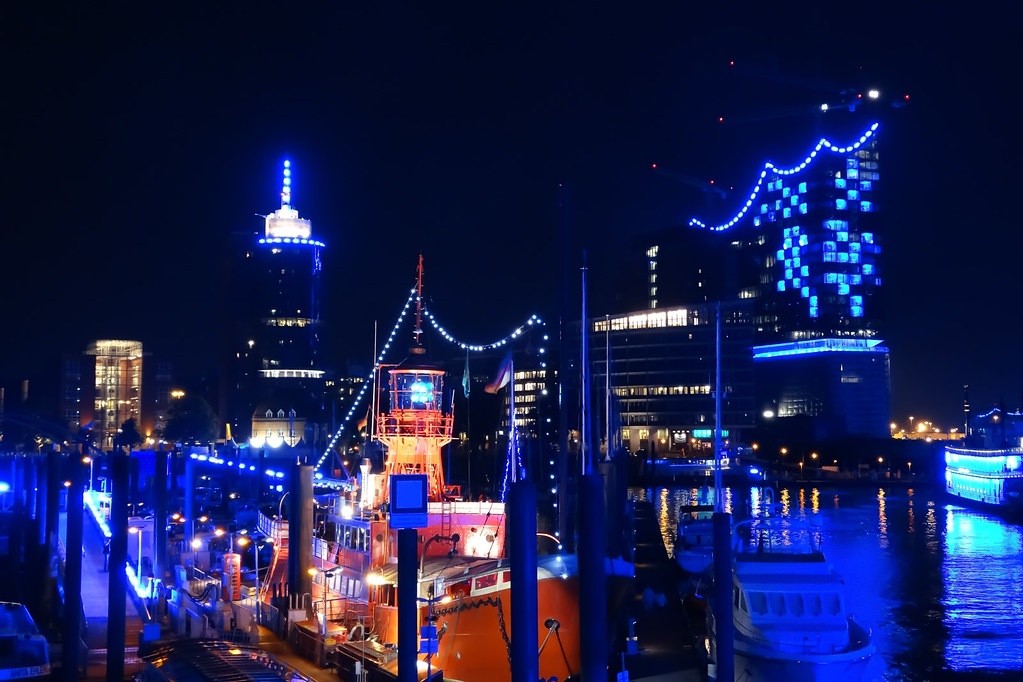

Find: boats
255;252;584;682
692;512;874;681
670;501;717;572
1;600;51;682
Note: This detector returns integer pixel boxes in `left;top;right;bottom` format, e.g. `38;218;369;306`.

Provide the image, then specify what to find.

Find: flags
484;347;513;394
462;357;470;399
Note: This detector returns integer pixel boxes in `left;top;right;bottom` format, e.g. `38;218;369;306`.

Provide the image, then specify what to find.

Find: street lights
215;529;249;603
237;537;275;625
129;527;142;585
83;456;94;493
128;503;144;516
306;565;343;634
172;512;208;580
